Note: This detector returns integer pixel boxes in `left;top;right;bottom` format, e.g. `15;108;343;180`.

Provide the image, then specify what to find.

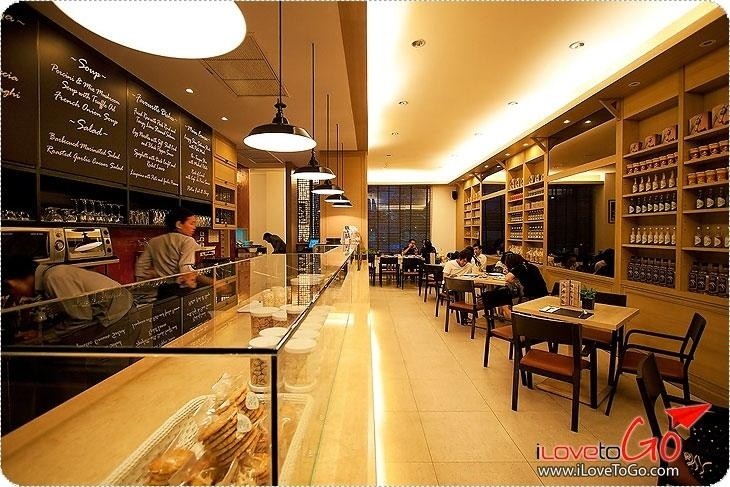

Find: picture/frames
608;200;615;223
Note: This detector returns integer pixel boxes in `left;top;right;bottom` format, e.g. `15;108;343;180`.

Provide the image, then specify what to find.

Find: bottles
216;186;232;204
624;168;678;290
435;253;440;264
247;283;318;393
682;186;729;301
215;208;235;225
508;171;544;265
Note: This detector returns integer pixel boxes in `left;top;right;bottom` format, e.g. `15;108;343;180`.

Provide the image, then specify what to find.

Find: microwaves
62;225;114;261
1;226;65;266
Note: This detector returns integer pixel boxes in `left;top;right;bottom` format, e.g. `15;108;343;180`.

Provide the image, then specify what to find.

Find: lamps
290;43;337;180
242;1;318;153
331;142;353;208
74;232;102;251
311;94;345;194
323;124;350;203
52;1;247;60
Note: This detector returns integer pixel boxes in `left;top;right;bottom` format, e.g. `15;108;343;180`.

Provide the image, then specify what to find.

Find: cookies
145;359;271;486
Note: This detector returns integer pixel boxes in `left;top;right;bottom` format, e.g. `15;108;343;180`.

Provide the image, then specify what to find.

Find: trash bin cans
326;238;341;244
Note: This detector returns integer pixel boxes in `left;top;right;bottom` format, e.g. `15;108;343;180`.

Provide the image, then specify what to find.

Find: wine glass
2;198;174;225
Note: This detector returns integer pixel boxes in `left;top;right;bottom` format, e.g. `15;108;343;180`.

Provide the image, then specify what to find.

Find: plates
487;272;503;276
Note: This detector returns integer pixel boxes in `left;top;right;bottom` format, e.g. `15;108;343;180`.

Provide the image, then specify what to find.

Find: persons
442;249;475;325
548;245;614;277
504;252;548;300
402;238;418;281
133;207;232;346
1;250;131;347
418;238;515;275
262;232;286;254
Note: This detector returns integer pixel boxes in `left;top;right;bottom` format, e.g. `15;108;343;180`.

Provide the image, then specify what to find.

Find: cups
429;252;436;264
193;214;212;227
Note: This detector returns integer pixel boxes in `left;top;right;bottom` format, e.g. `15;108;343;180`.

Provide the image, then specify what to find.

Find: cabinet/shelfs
212;129;237;230
